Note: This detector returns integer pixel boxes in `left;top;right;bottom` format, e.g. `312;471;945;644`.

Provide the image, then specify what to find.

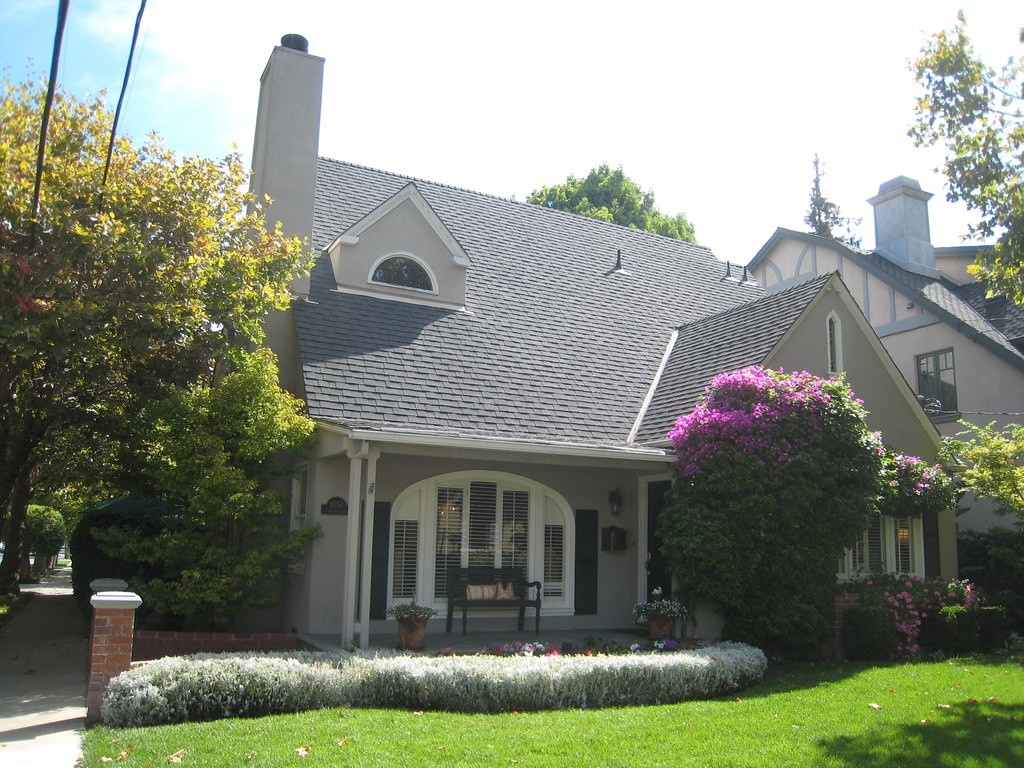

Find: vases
648;615;673;640
395;616;429;650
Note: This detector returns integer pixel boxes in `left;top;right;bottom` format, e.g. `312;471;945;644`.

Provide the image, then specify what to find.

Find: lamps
609;488;624;514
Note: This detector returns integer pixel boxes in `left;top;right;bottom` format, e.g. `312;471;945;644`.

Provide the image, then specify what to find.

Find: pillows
467;584;497;601
497;581;515;600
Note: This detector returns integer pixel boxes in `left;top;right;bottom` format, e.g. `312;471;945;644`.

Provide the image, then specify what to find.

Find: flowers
382;587;438;621
632;599;697;628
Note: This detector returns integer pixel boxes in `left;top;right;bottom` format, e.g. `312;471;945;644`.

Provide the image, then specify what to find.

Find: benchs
446;566;542;637
